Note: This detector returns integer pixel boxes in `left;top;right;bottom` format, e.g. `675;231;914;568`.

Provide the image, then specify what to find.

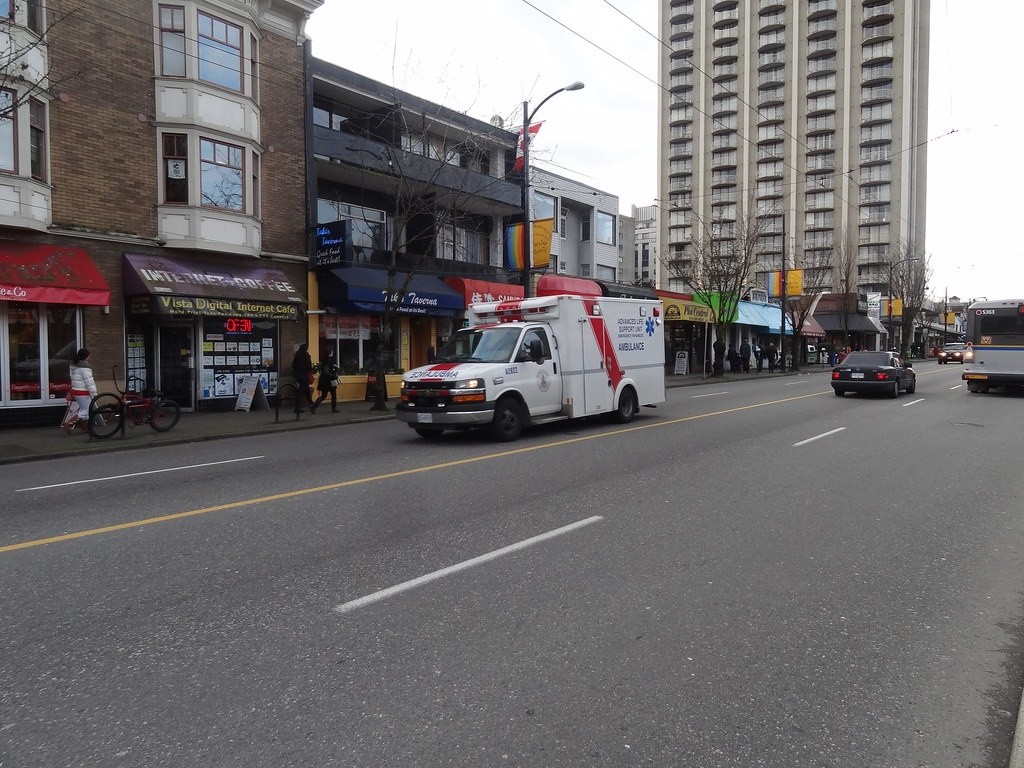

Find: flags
516;123;542;172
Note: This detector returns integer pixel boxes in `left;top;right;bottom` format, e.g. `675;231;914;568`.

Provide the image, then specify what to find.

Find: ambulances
396;275;668;442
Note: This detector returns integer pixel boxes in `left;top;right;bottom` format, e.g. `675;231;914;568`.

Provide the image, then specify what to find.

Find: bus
960;297;1024;399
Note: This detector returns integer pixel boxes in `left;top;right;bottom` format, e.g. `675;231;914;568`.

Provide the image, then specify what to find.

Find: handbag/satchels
66;392;74;401
307;371;314;384
331;377;341;387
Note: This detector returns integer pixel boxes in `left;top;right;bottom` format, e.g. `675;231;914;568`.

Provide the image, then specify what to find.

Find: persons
740;339;751;374
753;343;778;373
713;337;726;376
910;343;916;358
828;348;846;367
310;346;340;414
63;348;98;433
728;344;737;373
292;344;313;413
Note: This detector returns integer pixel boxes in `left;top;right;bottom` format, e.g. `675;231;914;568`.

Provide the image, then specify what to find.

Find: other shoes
332;409;340;412
80;421;89;433
62;421;73;435
294;408;305;413
310;405;315;415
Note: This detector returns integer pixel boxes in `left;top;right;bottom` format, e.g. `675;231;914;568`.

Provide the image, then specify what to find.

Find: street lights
520;80;595;300
890;257;920;351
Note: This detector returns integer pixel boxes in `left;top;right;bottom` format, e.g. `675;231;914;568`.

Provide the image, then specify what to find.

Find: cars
938;342;963;364
830;350;917;400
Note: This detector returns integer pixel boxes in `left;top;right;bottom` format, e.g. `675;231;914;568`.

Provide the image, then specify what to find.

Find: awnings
123;252;308;322
595;280;659;300
319;266;464;318
0;240;110;306
731;300;826;336
537;275;602;297
445;276;524;309
658;296;716;323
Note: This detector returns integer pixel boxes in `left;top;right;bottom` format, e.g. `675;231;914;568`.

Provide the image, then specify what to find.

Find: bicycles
87;365;181;439
